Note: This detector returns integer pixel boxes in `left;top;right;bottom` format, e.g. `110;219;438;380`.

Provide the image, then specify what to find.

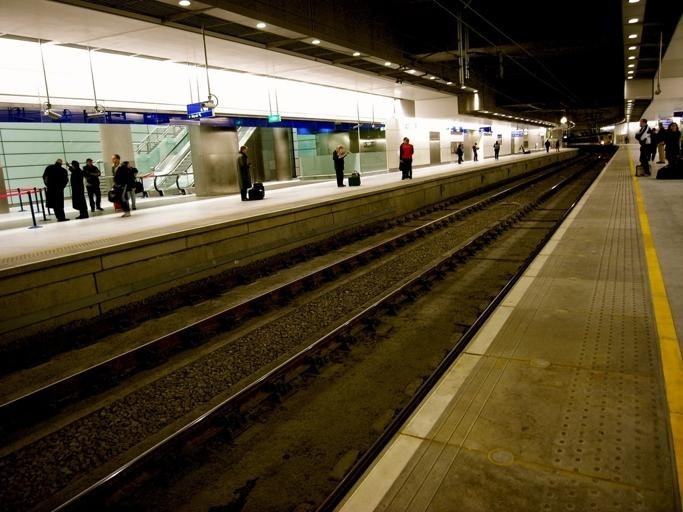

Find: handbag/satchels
135;182;143;194
107;185;129;202
349;176;360;186
247;183;264;202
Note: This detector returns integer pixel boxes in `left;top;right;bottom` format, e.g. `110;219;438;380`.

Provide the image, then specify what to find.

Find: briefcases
636;165;651;177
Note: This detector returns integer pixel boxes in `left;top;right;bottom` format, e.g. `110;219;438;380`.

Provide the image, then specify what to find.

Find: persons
493;141;499;159
42;159;70;222
554;139;559;152
635;118;652;177
109;153;139;218
544;140;549;152
235;146;252;201
82;158;104;211
647;127;656;162
664;122;680;163
456;144;462;164
65;160;90;219
332;145;347;187
399;137;410;179
400;139;413;180
623;136;627;144
471;142;478;161
655;122;665;163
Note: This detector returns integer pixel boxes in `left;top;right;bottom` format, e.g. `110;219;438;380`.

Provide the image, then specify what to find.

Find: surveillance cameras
86;113;104;118
202;102;216;108
48;110;62;120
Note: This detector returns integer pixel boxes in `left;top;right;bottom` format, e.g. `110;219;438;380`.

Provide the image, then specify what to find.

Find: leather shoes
57;213;90;222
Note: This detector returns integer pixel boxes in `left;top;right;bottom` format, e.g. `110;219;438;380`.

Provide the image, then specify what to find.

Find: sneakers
91;207;104;212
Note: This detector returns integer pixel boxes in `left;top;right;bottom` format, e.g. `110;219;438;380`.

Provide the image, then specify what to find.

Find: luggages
352;170;360;176
